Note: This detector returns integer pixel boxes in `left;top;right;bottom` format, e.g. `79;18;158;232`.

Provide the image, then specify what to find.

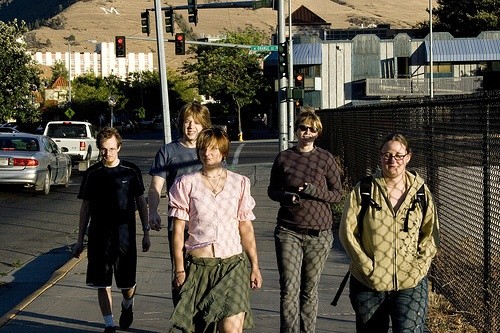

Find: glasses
296;124;318;133
380;152;410;160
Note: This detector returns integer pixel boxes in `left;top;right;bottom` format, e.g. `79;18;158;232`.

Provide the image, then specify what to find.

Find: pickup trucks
43;120;103;171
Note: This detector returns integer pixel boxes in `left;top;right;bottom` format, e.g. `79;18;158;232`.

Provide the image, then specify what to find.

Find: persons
73;125;151;333
267;112;343;333
168;126;263;333
142;225;150;231
148;102;211;307
339;133;439;333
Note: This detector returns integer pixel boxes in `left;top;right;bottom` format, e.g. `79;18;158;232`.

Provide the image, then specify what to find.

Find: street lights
68;39;97;102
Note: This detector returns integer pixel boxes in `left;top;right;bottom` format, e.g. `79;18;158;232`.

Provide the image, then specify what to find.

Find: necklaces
201;169;225;194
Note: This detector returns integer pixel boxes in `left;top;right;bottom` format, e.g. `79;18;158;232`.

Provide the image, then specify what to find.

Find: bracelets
174;270;185;273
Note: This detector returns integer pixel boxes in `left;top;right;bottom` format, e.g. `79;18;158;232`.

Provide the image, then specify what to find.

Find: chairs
26;142;37;150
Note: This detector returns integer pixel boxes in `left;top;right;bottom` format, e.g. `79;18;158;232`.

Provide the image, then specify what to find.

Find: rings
251;281;255;283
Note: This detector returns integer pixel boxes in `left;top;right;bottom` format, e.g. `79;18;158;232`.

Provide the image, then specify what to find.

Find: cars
0;131;73;194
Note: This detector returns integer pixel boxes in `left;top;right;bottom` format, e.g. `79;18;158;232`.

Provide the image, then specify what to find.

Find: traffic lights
175;33;186;55
140;12;150;36
295;74;305;107
115;36;126;57
164;9;174;37
187;0;198;23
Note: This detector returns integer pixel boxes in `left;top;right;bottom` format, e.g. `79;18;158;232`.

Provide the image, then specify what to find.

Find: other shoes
119;297;135;329
103;326;117;333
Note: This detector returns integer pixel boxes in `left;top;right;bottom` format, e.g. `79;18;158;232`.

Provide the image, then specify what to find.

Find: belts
277;219;323;237
